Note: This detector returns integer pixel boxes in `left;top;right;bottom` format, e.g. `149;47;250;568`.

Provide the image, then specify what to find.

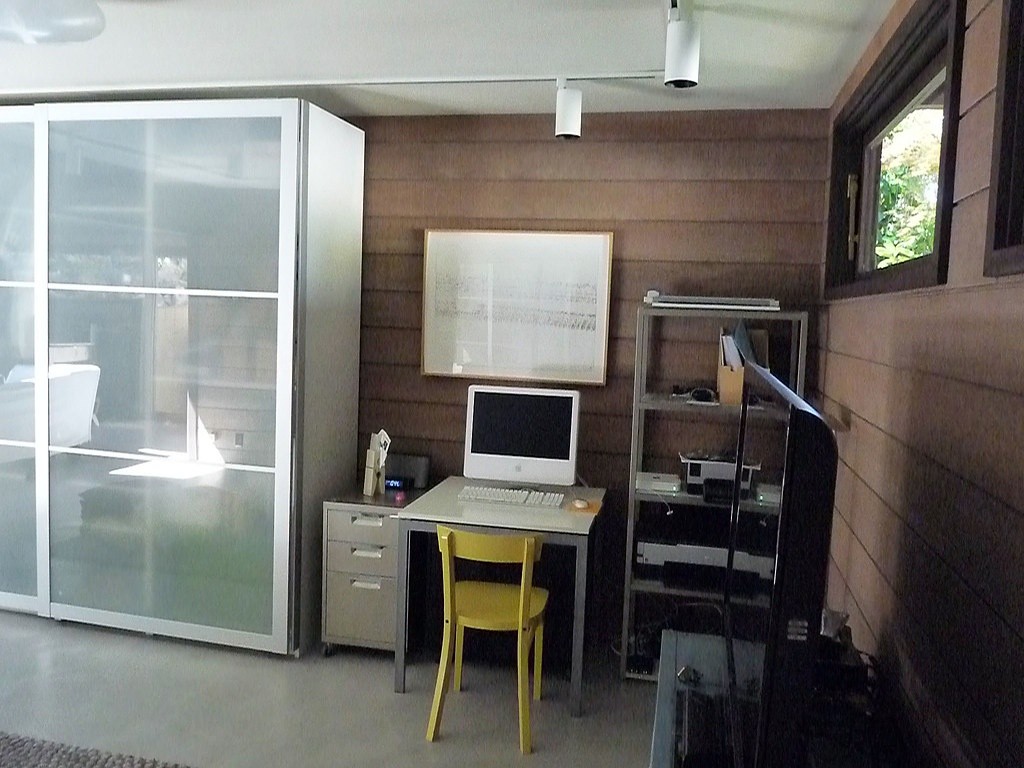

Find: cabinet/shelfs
0;98;366;658
621;298;807;683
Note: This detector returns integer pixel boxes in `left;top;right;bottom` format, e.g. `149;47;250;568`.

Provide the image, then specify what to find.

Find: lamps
665;0;701;89
555;77;582;138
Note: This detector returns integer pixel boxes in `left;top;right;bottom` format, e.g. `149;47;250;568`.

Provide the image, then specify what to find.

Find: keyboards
459;486;566;508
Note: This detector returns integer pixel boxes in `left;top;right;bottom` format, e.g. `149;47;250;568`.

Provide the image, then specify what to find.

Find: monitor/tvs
463;385;580;491
678;360;838;768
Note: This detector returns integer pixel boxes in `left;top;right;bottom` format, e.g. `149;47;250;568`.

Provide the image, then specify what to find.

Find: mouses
572;498;589;509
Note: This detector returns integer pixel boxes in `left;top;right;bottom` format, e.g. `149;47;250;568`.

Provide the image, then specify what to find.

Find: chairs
427;523;551;755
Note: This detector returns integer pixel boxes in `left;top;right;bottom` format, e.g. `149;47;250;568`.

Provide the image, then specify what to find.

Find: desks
648;630;729;768
323;485;608;717
